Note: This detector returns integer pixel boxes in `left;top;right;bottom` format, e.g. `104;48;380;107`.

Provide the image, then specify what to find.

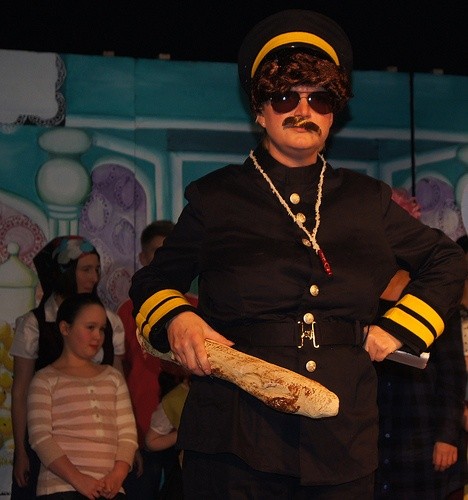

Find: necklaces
247;147;338;279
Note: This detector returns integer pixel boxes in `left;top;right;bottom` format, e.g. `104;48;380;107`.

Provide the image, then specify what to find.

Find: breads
136;326;340;420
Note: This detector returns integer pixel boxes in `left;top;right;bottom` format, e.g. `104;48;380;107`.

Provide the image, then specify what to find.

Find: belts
218;319;360;348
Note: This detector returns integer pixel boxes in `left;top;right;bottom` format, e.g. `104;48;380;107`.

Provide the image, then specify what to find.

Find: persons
143;371;195;469
114;219;203;500
376;189;468;500
10;234;128;500
125;9;468;500
25;291;138;500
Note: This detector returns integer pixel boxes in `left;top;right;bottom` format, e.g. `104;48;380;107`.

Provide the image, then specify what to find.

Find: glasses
261;90;339;115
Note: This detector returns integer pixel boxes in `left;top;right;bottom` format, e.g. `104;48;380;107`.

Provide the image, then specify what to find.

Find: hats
237;10;353;95
